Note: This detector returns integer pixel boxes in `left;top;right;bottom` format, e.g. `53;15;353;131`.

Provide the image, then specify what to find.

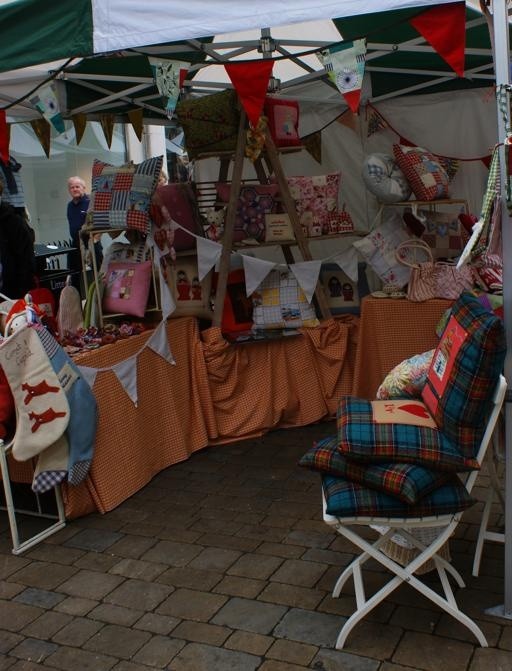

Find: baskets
369;523;452;576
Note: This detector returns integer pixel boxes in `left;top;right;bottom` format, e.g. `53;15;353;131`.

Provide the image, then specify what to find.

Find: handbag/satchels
396;239;470;302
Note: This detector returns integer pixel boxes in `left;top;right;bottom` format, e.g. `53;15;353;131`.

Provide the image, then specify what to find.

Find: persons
190;276;202;301
176;269;191;300
67;176;104;299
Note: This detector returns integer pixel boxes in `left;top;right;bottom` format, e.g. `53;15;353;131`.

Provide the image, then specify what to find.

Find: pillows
172;88;248;163
319;471;478;516
420;286;509;460
334;394;482;472
375;348;437;398
298;435;456;507
83;145;471;343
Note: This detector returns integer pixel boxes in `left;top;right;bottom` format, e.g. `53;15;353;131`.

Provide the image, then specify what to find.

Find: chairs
319;373;509;651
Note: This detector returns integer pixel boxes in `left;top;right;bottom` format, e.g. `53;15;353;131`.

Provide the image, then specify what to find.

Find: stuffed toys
205;206;226;239
245;115;269;162
9;312;27;336
